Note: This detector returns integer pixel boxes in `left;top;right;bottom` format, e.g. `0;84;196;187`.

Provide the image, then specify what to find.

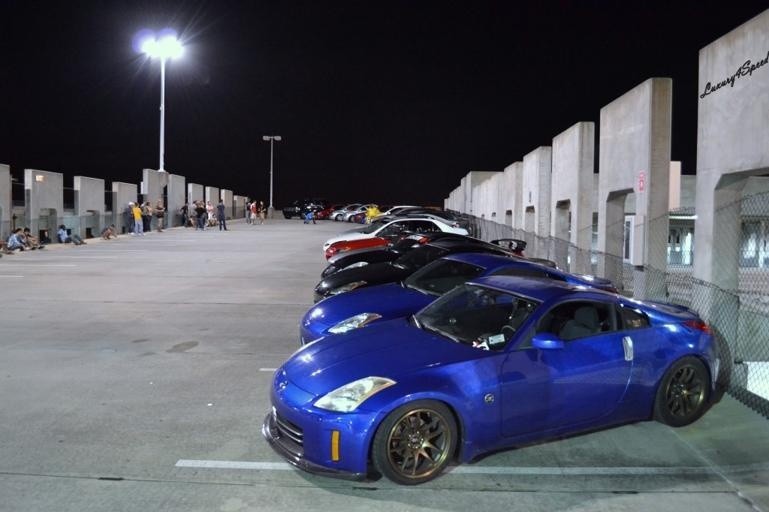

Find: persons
127;200;166;237
180;200;217;231
304;212;317;224
56;224;87;245
0;227;45;257
245;200;266;225
366;203;380;223
217;199;227;231
101;224;116;239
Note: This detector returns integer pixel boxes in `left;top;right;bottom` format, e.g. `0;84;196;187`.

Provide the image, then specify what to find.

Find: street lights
142;34;185;172
262;135;282;220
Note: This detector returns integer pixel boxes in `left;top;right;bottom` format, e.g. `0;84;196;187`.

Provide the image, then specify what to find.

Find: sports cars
260;272;722;484
312;238;558;305
300;251;619;350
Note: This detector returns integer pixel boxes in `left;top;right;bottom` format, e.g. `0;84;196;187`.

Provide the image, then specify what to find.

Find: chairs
560;306;601;339
511;308;556;332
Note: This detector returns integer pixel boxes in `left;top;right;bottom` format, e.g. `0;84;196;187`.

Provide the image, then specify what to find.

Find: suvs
281;195;331;219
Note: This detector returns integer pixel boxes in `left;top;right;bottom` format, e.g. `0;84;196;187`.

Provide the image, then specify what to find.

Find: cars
316;200;526;279
